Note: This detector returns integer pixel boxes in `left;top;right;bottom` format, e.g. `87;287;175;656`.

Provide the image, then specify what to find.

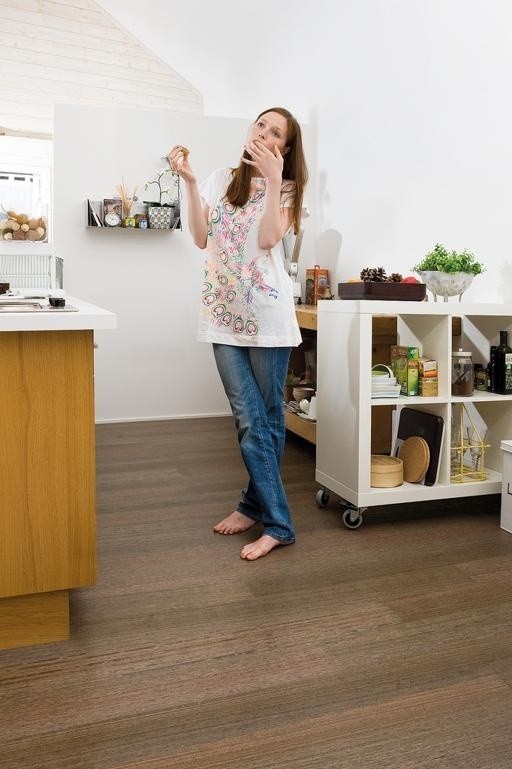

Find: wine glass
467;426;489;477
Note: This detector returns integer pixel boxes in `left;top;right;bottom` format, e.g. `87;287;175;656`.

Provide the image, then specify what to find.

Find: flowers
144;169;178;208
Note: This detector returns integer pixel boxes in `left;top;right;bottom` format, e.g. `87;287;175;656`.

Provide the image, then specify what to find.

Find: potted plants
338;265;427;301
408;240;489;302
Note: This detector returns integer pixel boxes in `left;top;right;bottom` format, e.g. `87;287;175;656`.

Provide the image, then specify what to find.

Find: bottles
486;330;512;395
451;348;475;397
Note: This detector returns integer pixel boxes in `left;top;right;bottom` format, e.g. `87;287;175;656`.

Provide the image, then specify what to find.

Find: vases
147;206;172;230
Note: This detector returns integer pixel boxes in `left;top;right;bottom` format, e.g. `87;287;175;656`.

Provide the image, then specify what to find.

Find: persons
167;106;309;562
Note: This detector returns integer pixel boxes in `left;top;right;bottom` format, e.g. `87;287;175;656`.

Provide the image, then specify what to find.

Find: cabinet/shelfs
2;331;100;651
283;304;317;446
314;302;511;531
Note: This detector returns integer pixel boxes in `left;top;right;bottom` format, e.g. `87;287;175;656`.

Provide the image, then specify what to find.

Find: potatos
7;211;46;241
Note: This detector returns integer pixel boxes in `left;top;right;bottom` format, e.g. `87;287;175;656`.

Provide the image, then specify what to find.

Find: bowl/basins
293;387;315;404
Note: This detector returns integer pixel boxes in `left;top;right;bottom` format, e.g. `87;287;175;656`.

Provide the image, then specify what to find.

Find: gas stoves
0;298;79;314
